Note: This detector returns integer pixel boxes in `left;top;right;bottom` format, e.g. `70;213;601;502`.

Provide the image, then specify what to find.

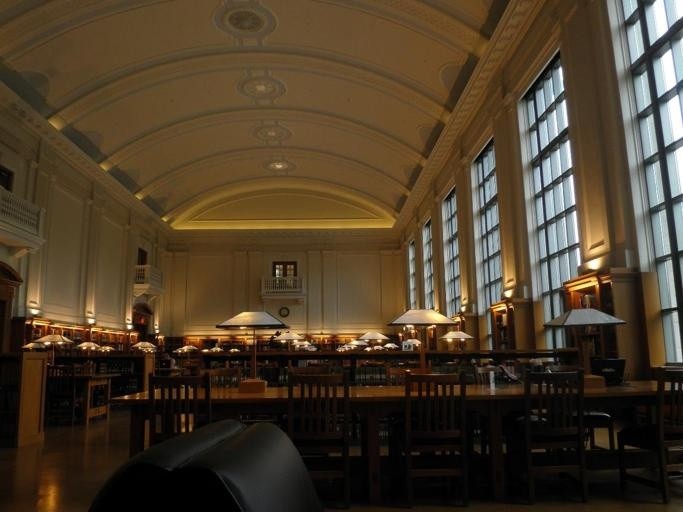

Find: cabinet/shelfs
556;267;650;380
488;297;536;351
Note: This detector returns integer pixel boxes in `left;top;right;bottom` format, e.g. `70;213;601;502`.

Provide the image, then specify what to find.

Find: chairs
86;419;247;511
178;423;323;511
510;368;587;505
147;373;211;445
389;371;470;507
283;372;351;506
47;348;683;429
618;367;682;504
